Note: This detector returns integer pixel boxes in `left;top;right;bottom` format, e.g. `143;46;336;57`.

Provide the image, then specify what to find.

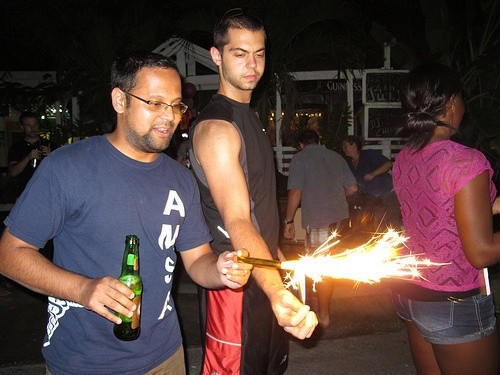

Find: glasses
123;91;188;115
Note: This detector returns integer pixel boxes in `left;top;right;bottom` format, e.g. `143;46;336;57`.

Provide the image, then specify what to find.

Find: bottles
185;149;191;169
31;140;40;168
114;235;143;341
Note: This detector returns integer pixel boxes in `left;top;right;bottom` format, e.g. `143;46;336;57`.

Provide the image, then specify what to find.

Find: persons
283;131;358;329
342;135;400;228
7;113;58;196
189;9;318;375
392;63;500;375
177;138;191;168
0;52;252;375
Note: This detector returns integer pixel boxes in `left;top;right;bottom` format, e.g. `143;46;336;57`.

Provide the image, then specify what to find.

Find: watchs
284;216;293;224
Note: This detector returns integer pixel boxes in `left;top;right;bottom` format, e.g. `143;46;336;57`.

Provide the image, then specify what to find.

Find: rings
244;270;247;276
